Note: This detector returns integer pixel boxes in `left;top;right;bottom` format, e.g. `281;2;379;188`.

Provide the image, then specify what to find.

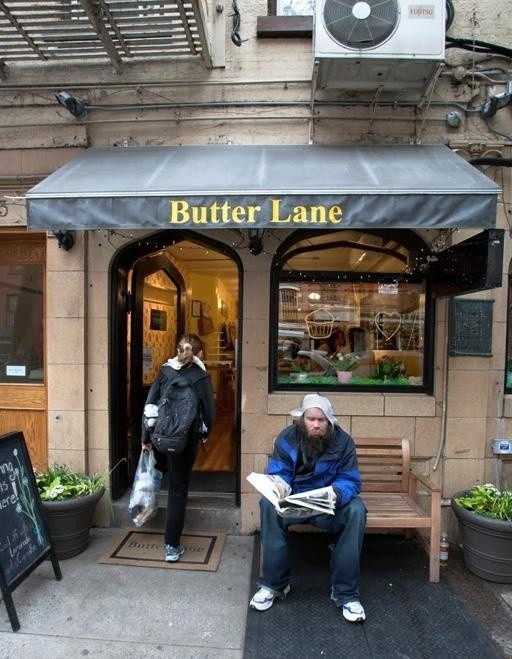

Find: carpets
97;526;226;572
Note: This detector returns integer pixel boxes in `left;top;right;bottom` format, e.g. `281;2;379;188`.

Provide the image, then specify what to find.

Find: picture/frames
192;300;201;317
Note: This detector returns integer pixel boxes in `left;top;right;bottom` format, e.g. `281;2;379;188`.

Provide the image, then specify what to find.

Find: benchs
259;438;440;584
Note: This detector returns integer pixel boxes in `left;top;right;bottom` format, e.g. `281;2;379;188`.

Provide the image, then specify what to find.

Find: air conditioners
312;2;445;89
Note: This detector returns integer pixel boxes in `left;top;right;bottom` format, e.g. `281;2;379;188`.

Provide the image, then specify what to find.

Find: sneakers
163;543;186;563
249;583;291;611
329;588;366;624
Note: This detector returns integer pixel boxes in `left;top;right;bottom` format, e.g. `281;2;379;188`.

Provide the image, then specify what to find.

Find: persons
249;394;367;624
140;334;216;562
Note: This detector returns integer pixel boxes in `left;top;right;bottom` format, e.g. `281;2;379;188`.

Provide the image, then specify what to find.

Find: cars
277;340;422;378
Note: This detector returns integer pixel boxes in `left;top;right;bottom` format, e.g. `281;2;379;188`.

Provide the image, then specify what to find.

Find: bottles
440;532;450;566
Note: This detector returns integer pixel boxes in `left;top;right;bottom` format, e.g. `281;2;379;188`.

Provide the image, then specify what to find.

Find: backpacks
147;363;211;457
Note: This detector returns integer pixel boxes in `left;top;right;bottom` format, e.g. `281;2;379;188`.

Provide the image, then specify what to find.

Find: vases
337;371;352;384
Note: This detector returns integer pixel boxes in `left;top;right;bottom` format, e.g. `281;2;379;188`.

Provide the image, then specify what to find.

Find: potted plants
451;484;512;583
33;458;126;560
289;360;310;381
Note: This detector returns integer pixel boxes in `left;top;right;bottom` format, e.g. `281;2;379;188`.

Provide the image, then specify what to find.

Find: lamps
55;92;84;116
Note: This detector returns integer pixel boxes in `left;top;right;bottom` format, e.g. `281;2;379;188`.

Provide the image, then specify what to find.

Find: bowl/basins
492;438;512;455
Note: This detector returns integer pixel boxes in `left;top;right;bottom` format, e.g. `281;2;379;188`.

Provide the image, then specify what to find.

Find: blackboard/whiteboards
0;431;53;590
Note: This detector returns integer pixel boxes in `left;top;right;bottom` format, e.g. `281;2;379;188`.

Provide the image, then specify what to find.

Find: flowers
331;354;359;371
